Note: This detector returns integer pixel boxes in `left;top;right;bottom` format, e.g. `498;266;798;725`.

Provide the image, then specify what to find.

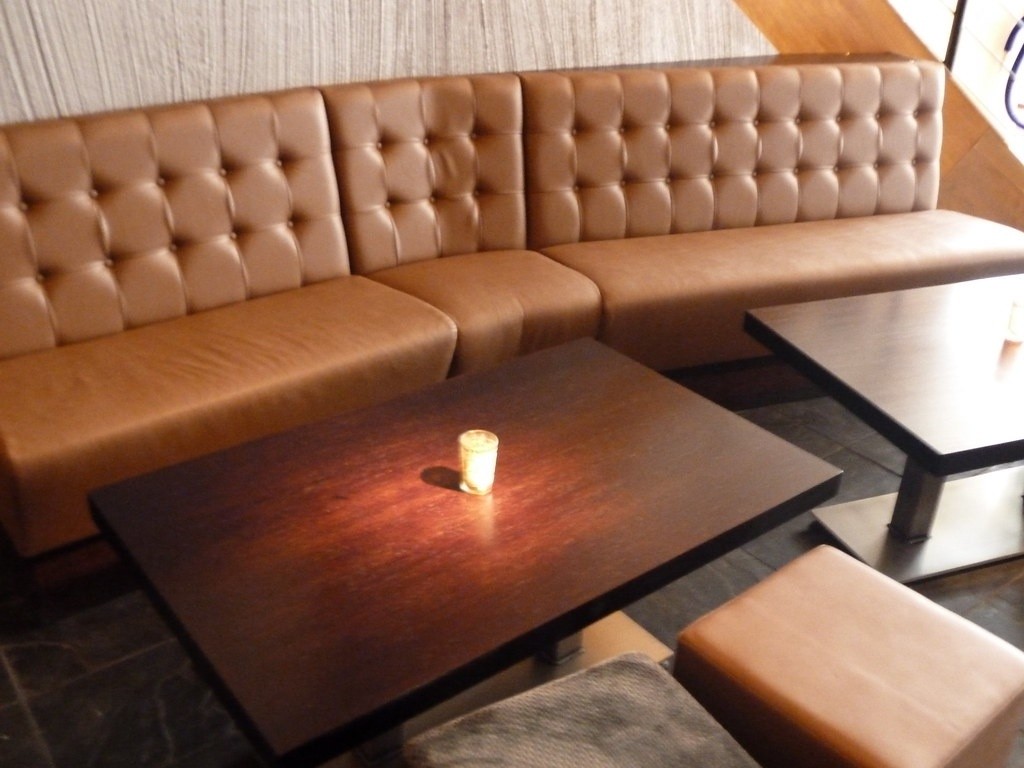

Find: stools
408;654;765;768
675;545;1024;767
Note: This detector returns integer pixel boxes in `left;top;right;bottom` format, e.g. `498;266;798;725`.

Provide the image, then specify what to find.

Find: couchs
0;55;1024;554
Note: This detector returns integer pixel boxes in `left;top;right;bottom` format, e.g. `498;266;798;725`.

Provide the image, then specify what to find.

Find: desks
745;273;1024;585
87;336;841;768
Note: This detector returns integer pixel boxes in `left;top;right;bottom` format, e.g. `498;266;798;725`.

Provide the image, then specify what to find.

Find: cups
1004;302;1024;344
458;431;499;494
